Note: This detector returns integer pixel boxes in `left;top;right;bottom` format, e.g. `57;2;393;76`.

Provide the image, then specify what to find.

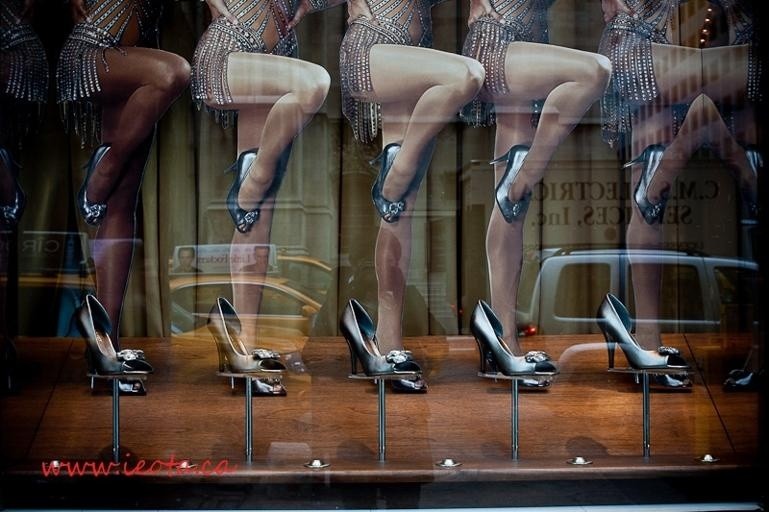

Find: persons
648;0;769;392
1;0;49;401
55;0;193;358
189;0;347;355
338;2;490;356
610;1;765;351
461;0;612;359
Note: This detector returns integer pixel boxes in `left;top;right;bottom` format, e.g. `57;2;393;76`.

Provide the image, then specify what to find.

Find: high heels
743;148;765;229
595;293;692;369
470;299;558;376
635;371;689;389
721;346;761;393
390;378;426;393
207;297;289;373
0;147;28;229
339;298;423;374
230;376;287;396
90;376;146;397
518;376;555;393
78;143;114;229
488;146;529;224
370;144;404;223
621;142;665;225
73;294;154;377
223;150;260;233
